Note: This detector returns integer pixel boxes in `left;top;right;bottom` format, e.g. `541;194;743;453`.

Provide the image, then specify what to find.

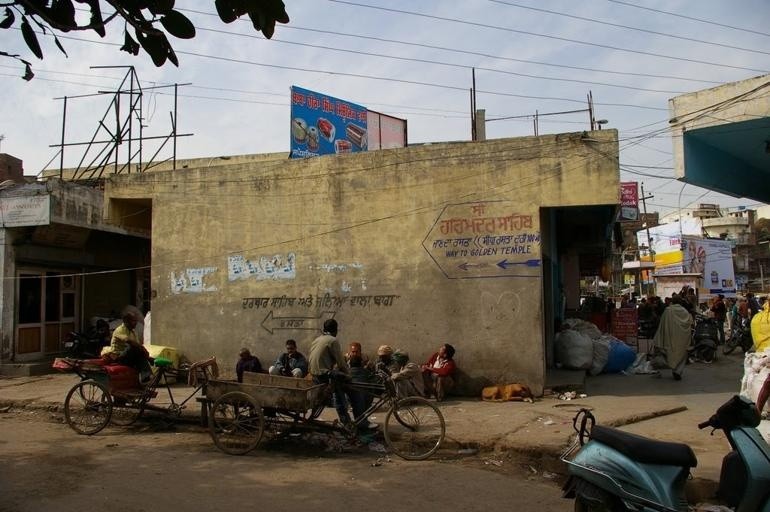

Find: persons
269;339;308;379
621;285;770;346
390;349;425;401
236;348;261;383
420;344;457;402
368;344;399;378
309;319;379;429
111;311;154;383
334;343;369;370
688;242;706;280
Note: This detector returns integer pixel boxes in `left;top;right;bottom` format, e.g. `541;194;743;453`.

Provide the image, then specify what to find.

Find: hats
391;352;409;363
377;344;393;356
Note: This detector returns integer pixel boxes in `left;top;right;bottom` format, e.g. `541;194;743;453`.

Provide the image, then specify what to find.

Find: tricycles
53;348;218;437
199;361;447;462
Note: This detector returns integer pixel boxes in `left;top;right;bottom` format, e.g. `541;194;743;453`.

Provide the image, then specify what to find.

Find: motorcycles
62;318;110;358
689;312;719;363
561;396;770;512
724;313;751;355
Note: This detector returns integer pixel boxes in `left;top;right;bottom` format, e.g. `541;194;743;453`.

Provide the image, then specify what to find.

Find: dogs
480;382;535;404
